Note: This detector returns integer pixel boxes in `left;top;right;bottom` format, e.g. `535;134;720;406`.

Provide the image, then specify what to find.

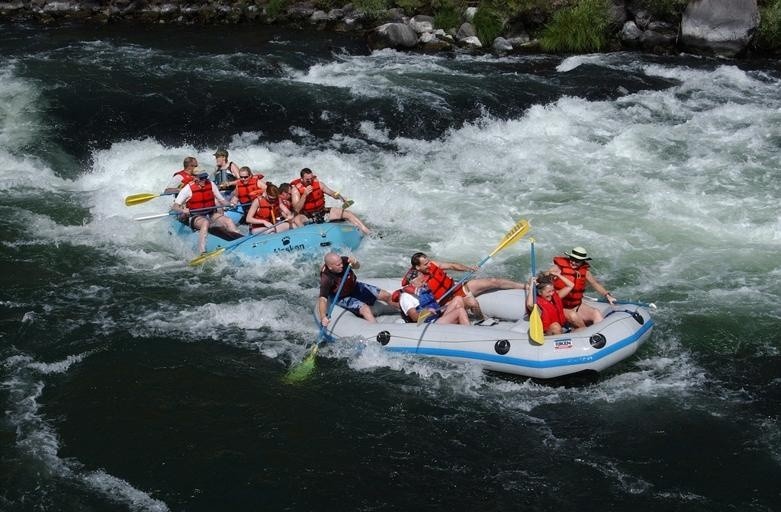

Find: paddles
125;192;175;207
435;220;530;303
417;304;447;326
190;219;289;267
528;236;544;345
283;262;352;383
334;191;354;210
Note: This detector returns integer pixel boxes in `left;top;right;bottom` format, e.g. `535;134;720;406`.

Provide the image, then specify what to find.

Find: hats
212;148;229;157
564;246;592;261
192;167;209;178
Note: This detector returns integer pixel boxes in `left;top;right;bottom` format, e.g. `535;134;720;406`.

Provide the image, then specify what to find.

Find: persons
162;148;374;258
402;253;529;320
319;253;402;327
392;270;471;326
524;267;586;336
546;246;617;328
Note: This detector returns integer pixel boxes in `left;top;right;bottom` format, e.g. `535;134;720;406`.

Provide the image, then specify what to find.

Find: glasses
240;175;249;179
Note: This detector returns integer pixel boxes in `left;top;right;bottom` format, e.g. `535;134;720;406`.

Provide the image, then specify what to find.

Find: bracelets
604;292;611;298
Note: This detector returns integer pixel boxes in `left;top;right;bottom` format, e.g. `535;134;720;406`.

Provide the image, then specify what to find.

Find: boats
167;200;370;266
312;276;657;382
